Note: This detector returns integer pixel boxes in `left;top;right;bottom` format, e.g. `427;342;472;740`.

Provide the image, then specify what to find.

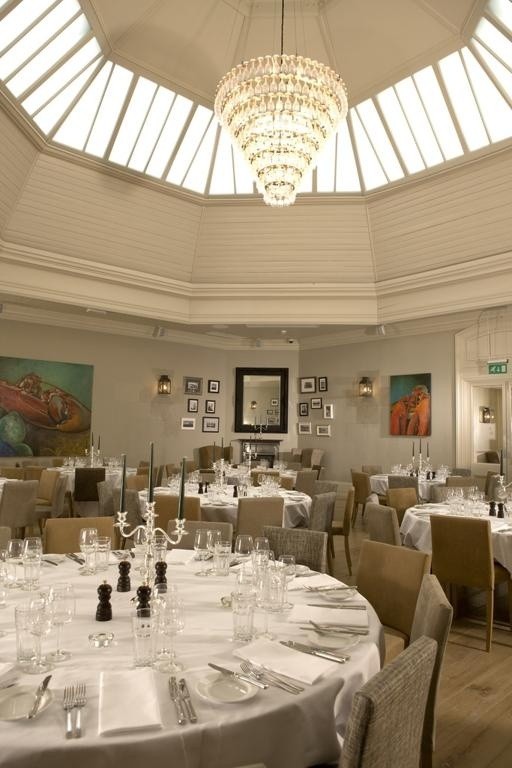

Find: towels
230;635;340;686
96;667;163;736
0;662;19;689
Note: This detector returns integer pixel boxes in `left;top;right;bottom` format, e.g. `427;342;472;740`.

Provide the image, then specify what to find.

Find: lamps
358;375;373;397
213;0;350;206
157;374;171;394
250;399;258;409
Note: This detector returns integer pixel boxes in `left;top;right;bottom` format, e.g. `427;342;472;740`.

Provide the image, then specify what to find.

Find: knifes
128;546;136;558
207;662;269;689
281;639;350;663
28;675;53;720
170;675;186;724
70;552;85;562
44;559;58;566
178;678;198;722
66;553;84;566
308;602;369;610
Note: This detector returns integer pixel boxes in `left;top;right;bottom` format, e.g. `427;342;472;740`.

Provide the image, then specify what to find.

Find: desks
400;499;512;608
370;470;460;498
136;478;313;524
1;546;385;768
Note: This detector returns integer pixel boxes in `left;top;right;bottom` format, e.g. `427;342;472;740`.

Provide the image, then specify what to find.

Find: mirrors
234;366;288;433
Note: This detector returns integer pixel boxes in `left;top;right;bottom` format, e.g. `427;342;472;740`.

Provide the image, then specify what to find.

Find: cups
231;592;254;644
23;548;43;579
14;602;42;668
213;540;231;576
132;608;157;664
95;535;111;571
252;536;271;567
156;536;167;562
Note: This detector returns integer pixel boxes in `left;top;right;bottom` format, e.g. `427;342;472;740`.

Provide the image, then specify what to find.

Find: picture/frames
201;415;220;433
266;397;280;424
299;401;309;416
205;399;216;413
187;398;199;412
298;421;312;434
318;376;328;391
323;403;334;419
180;416;196;430
182;375;203;395
207;378;220;393
316;424;332;437
299;375;317;394
311;396;323;409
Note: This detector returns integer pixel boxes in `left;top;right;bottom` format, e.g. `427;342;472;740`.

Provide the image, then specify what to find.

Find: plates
0;685;55;721
195;673;259;704
322;586;353;602
309;626;360;651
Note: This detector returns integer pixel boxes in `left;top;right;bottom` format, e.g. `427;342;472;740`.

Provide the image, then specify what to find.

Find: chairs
71;467;106;502
1;443;472;487
36;470;60;505
166;518;234;553
364;501;403;544
350;468;372;527
446;477;476;487
408;571;456;766
431;487;479;504
37;475;67;521
427;513;512;653
386;487;417;523
237;497;285;537
45;518;117;554
354;538;433;671
262;525;330;572
386;474;420;499
109;486;147;550
0;480;40;534
153;491;201;530
306;491;336;534
296;471;318;494
335;634;441;767
333;486;357;576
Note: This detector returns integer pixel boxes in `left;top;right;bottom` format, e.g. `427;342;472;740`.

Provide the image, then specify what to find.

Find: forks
240;658;303;695
303;584;359;592
300;620;369;636
63;686;77;738
74;681;87;737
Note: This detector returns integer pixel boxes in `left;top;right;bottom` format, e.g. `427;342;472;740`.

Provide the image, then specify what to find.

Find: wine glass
193;528;211;577
134;529;147;572
163;470;281;503
47;582;75;663
445;482;510;518
80;531;97;576
255;576;283;640
5;538;24;589
233;535;254;584
24;596;53;674
389;461;449;483
207;530;221;573
234;567;253;614
277;554;296;608
60;454;122;473
255;550;276;608
220;457;289;473
153;583;177;661
162;594;186;676
77;528;98;571
20;537;42;592
